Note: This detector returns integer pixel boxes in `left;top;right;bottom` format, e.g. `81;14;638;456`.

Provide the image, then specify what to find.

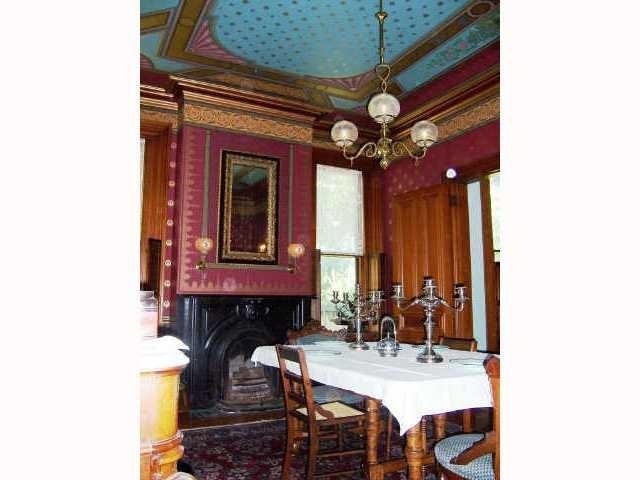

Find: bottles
140;290;159;342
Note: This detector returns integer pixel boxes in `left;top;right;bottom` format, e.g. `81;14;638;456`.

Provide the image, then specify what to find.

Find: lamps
326;1;438;171
192;236;213;270
283;245;306;272
330;276;465;361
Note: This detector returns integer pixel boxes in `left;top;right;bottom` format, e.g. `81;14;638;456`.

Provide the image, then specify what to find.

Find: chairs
432;354;500;479
439;335;478;434
274;343;365;480
287;318;354;344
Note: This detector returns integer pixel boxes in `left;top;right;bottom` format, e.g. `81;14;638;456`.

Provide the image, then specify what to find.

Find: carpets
181;413;461;479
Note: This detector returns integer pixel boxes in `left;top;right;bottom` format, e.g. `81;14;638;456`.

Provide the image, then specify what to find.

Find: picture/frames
215;148;280;266
